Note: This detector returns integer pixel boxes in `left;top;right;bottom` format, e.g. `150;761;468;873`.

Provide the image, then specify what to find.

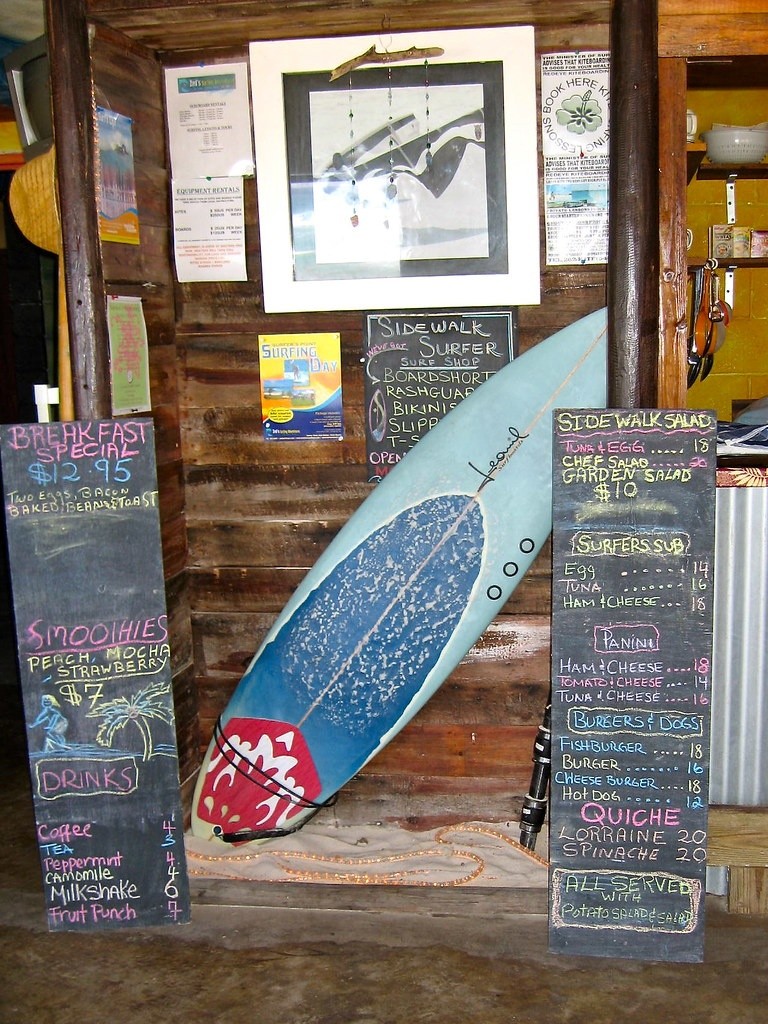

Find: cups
686;108;698;144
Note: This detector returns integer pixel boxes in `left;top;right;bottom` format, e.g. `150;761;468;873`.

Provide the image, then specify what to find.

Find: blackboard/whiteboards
0;414;199;940
358;302;523;485
549;406;720;968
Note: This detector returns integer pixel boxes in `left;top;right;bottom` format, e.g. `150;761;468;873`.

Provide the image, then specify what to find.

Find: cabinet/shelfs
656;0;768;462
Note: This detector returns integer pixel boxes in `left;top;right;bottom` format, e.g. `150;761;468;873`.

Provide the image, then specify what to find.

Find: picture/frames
249;25;541;314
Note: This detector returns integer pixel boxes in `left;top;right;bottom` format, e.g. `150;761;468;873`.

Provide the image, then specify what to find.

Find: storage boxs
708;225;768;258
